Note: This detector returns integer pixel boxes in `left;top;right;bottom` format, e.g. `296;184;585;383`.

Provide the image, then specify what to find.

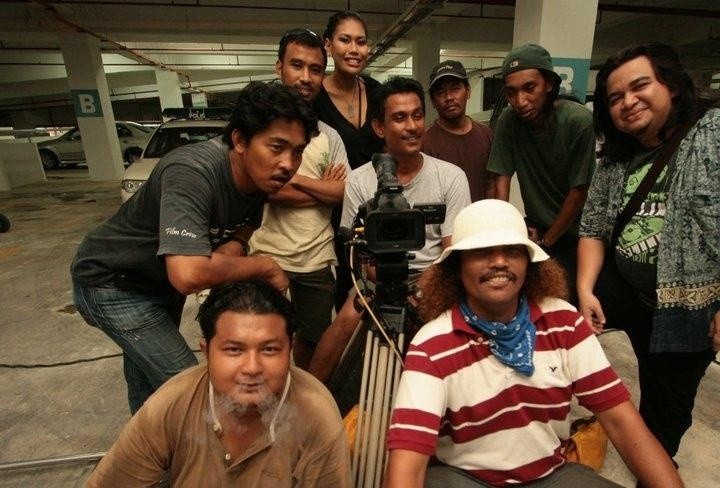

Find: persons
244;26;353;372
485;42;597;311
79;277;353;488
421;58;496;205
318;12;385;315
576;41;719;488
69;79;320;416
310;76;472;384
382;197;685;488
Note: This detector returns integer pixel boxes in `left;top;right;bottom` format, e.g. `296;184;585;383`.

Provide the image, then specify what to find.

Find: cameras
347;151;447;256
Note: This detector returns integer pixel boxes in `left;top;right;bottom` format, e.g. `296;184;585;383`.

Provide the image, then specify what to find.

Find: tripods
330;262;423;487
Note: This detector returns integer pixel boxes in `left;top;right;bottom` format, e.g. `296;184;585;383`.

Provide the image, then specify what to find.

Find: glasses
280;28;319;39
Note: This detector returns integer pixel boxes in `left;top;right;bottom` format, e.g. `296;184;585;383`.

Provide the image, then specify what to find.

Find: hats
426;60;467;90
432;198;551;268
501;43;562;86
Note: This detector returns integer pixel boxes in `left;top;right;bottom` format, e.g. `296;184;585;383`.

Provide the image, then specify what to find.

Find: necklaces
335;83;358;117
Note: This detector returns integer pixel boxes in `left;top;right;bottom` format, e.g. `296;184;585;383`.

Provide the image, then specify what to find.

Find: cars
37;122;155;170
119;117;230;204
